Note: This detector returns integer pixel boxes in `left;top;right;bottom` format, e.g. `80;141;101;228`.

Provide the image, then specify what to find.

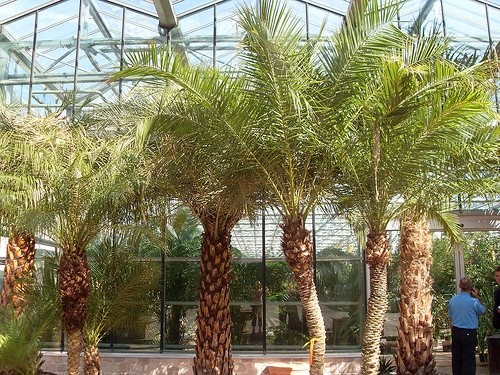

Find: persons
249;281;270;334
447;277;487;375
491;264;500;331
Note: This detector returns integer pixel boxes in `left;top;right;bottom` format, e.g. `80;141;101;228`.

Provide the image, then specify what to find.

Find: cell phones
472;286;476;294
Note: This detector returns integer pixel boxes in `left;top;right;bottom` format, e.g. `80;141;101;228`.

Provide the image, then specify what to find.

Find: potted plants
477;316;488;362
